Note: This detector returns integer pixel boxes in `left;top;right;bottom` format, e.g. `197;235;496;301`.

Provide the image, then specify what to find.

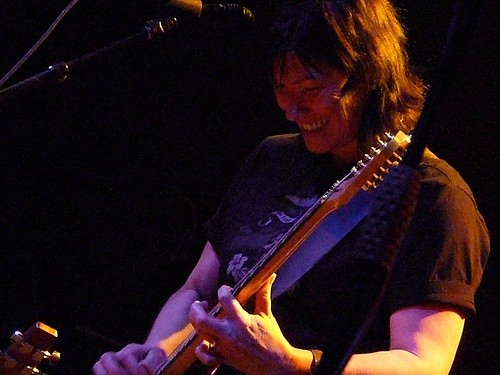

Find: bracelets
308;349;324;375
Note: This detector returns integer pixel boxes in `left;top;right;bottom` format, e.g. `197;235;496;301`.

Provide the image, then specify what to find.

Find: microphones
164;0;255;44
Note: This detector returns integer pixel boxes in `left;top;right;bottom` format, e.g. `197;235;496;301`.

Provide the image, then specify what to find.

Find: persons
92;0;490;375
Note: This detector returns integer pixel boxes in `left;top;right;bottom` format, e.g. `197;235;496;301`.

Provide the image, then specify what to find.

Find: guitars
0;322;60;375
152;130;412;375
